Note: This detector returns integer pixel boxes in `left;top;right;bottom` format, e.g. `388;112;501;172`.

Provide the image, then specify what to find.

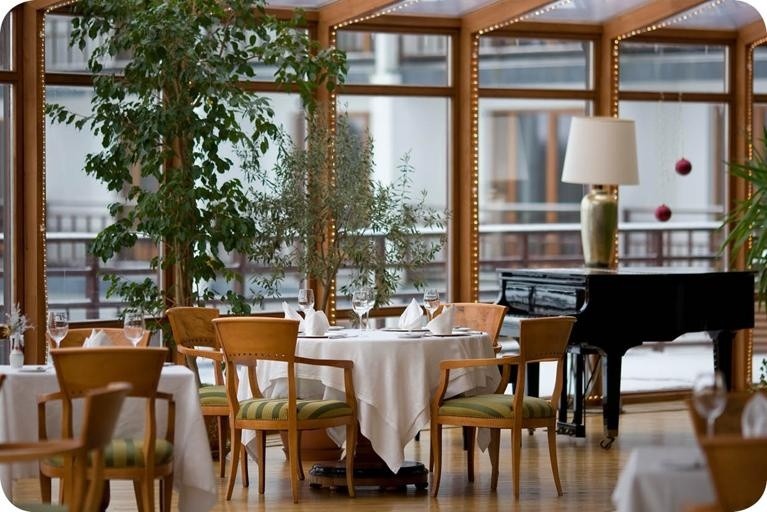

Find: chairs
167;307;249;487
37;347;175;512
427;301;508;482
431;317;577;499
212;317;357;503
684;393;754;440
48;328;151;347
700;436;767;510
0;382;133;512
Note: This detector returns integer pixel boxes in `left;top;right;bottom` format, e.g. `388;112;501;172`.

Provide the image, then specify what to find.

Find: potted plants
242;106;451;453
41;0;348;460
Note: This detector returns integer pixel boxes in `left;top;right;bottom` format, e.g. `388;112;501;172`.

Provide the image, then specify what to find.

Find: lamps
561;116;639;271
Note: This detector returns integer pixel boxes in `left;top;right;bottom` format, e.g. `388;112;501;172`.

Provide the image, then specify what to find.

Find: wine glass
359;287;375;335
693;371;729;435
423;288;440;319
123;312;146;347
47;311;69;348
298;288;315;311
351;291;370;336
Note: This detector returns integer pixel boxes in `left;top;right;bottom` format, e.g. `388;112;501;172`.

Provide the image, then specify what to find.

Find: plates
382;328;429;332
432;332;469;337
397;331;425;338
297;334;330;338
330;326;345;329
453;327;471;332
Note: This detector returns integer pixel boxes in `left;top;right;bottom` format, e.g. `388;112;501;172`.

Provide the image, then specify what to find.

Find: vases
9;333;24;368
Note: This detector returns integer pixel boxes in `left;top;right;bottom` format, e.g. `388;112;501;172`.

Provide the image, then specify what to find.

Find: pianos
491;267;754;451
